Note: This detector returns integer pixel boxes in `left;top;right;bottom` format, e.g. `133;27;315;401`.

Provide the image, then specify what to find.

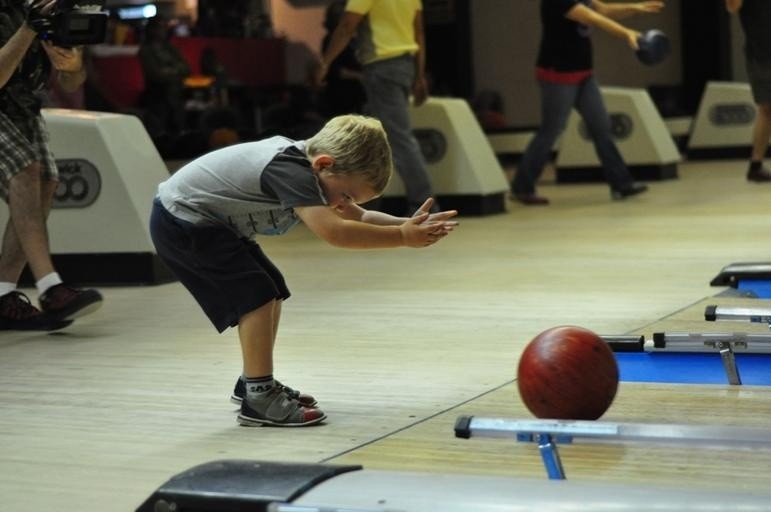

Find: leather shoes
235;387;328;428
232;375;317;406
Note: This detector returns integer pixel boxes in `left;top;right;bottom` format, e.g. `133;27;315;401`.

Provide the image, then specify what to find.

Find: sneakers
609;182;650;202
0;288;75;332
746;160;771;183
39;281;105;331
508;190;551;206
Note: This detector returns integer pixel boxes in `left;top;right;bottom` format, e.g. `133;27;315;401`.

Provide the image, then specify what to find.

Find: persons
725;1;770;181
1;1;104;333
501;1;670;207
313;0;444;218
149;113;459;429
